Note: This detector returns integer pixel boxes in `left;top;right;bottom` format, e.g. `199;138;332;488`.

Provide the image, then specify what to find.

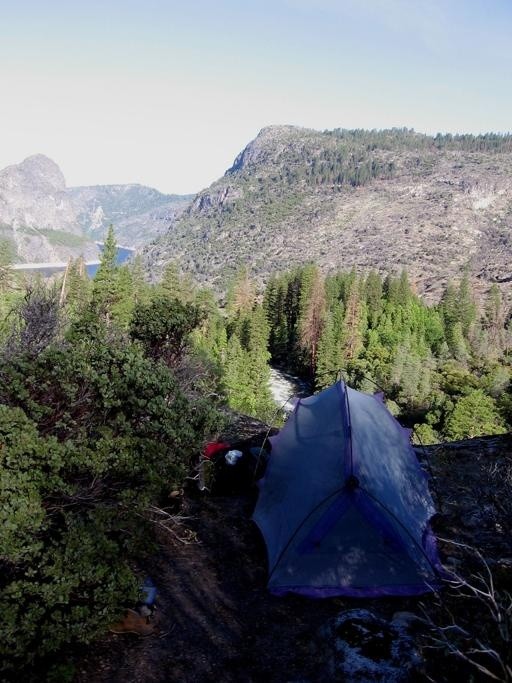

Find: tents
251;369;446;601
